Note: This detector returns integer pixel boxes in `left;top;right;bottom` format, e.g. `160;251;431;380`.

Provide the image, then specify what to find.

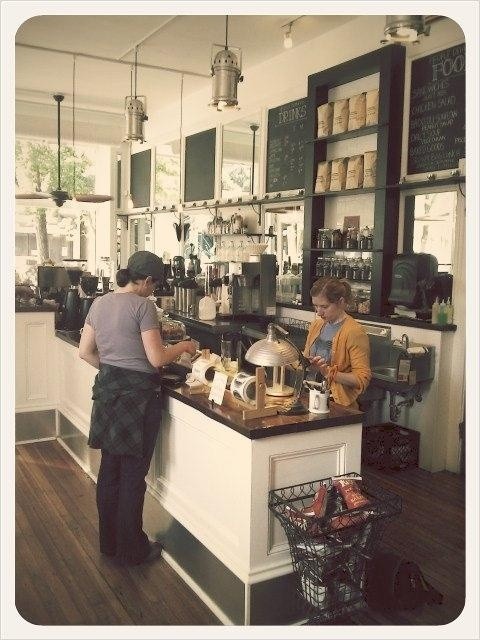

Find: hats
127;252;171;291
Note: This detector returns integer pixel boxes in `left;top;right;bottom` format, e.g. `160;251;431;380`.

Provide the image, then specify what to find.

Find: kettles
219;332;255;375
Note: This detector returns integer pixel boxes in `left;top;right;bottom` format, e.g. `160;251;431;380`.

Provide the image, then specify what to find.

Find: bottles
216;240;255;261
314;257;374;279
314;226;374;250
433;294;454;327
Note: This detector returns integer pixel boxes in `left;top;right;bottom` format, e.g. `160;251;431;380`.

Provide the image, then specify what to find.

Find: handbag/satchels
371;548;429;611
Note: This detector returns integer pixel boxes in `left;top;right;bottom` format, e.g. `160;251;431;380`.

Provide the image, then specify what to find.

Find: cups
308;390;329;412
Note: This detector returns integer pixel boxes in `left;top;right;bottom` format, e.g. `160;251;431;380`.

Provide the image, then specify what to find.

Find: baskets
362;422;420;475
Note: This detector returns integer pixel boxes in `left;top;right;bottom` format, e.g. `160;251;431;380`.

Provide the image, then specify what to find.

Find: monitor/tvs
37;267;70;290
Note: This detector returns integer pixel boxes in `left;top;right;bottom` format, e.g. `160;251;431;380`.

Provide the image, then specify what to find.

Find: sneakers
103;541;163;566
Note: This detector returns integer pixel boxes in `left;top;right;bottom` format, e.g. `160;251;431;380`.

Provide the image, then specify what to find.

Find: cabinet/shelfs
287;57;393;315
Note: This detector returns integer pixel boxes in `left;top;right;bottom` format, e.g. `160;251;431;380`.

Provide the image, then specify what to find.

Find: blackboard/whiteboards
400;40;467;182
180;122;222;207
266;97;306;196
128;146;155;211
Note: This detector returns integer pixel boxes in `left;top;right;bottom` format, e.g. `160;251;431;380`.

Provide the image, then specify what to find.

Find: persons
288;276;372;409
79;250;197;566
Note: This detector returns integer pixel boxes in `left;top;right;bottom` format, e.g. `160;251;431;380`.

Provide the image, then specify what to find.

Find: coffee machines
229;255;277;321
80;275;100;326
58;258;85;334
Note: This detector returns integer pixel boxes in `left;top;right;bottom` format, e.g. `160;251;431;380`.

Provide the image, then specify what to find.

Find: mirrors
150;136;181;207
216;107;264;200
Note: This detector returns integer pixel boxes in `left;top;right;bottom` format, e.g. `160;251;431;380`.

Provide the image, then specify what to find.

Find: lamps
123;47;149;143
66;55;82;217
283;20;293;49
381;15;421;45
43;94;70;206
209;16;244;111
